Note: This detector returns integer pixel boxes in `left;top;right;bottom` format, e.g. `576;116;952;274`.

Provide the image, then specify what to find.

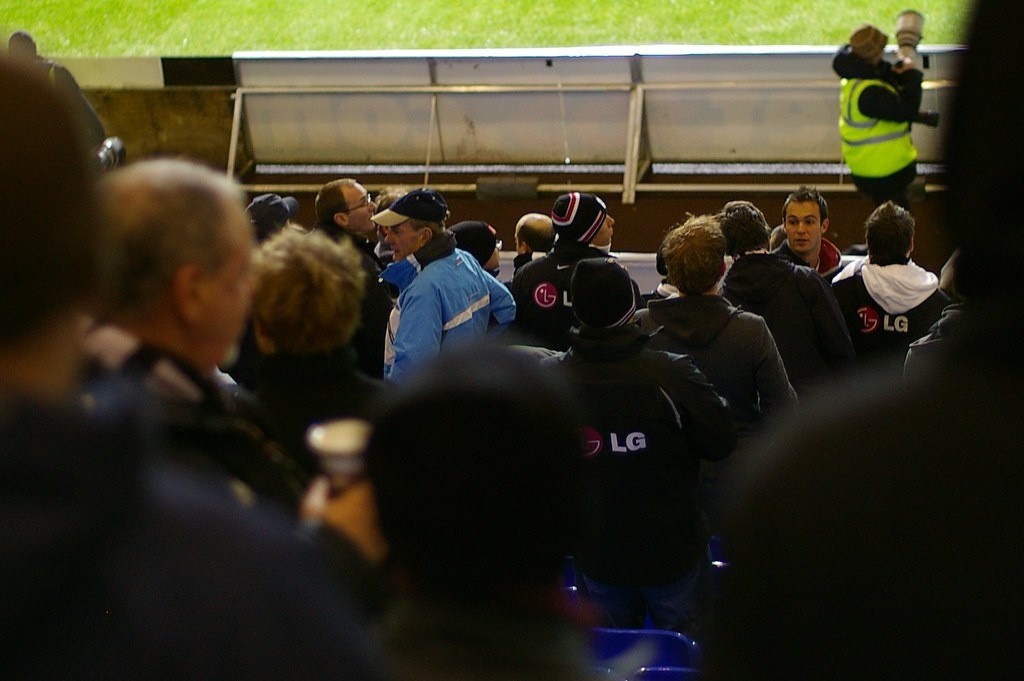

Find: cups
309;420;369;494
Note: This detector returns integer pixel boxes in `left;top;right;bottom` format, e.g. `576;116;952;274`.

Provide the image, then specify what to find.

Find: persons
0;0;1024;681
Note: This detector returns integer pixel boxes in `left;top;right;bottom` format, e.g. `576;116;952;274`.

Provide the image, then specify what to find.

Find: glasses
349;192;371;210
496;238;504;250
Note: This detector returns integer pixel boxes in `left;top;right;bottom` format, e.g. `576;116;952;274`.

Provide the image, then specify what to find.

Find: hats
849;24;889;61
569;257;637;328
550;191;607;246
370;187;449;227
243;193;299;230
447;220;497;267
